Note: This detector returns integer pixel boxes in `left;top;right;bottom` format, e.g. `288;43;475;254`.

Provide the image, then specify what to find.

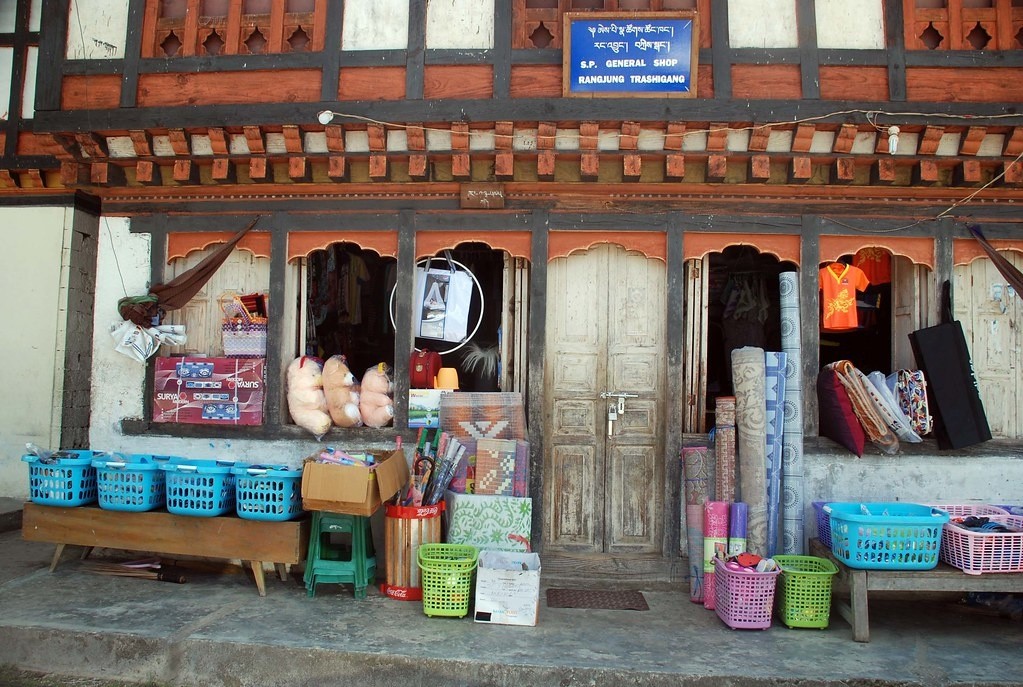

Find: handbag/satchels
409;349;442;389
414;250;473;342
907;279;992;451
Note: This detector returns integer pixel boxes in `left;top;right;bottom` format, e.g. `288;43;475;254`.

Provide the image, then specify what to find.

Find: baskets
415;543;478;619
770;554;839;629
810;500;1023;576
219;293;269;358
21;448;101;508
232;462;303;522
90;453;180;512
710;556;780;631
158;459;238;516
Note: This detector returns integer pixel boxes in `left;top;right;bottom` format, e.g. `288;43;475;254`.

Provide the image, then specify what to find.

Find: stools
303;510;378;600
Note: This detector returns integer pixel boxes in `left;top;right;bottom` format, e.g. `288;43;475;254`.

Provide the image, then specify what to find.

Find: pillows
815;367;866;458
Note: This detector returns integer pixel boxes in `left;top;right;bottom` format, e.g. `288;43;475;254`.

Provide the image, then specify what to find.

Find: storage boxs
408;388;454;428
473;549;541;627
445;489;532;575
301;447;410;518
153;356;267;426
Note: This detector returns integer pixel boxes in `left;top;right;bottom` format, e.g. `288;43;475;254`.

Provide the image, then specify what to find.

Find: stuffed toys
286;357;332;435
360;362;394;428
323;355;363;426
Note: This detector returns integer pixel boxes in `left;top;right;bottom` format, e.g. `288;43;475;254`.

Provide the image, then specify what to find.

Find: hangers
826;258;846;266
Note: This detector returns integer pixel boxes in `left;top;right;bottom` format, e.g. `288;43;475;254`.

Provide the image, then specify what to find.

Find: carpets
545;588;650;611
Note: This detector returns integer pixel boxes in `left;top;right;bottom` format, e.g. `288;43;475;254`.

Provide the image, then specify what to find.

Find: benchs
808;537;1023;644
21;499;312;597
